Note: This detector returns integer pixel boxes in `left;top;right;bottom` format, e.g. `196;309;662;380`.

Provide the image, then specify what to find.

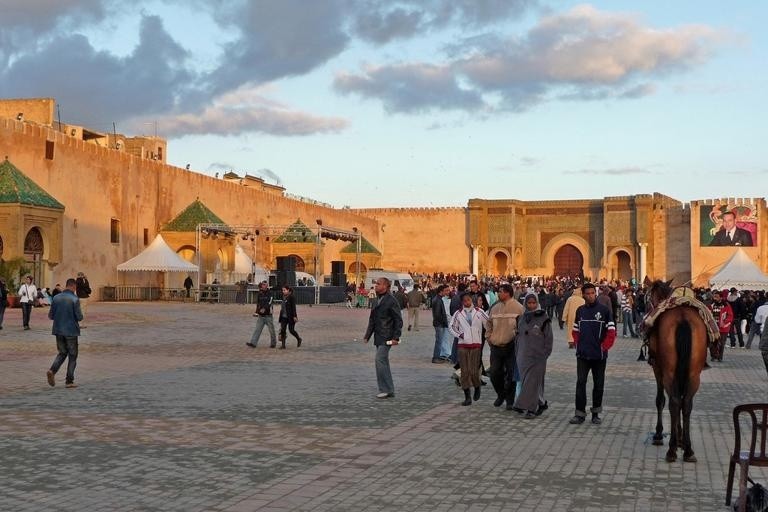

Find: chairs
725;403;768;512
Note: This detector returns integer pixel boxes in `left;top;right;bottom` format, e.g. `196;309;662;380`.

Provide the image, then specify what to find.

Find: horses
644;274;708;463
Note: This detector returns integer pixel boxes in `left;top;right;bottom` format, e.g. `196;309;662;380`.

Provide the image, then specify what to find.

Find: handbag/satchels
27;300;34;306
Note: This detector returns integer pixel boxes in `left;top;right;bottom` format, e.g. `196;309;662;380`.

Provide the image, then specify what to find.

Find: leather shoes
375;392;394;398
568;416;584;425
535;400;548;417
65;384;78;388
525;411;535;419
591;416;601;425
47;370;55;386
246;342;256;348
432;357;445;364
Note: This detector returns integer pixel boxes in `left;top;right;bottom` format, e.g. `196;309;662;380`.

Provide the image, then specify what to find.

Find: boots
289;329;301;347
461;388;471;406
493;386;503;407
278;330;286;349
472;386;480;401
504;387;513;410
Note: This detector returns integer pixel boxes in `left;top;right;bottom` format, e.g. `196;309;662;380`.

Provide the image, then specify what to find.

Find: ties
727;232;730;241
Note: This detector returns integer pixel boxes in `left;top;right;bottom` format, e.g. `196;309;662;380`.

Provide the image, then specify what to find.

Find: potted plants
0;256;39;308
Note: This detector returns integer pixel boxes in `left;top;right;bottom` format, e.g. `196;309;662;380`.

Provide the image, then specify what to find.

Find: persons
394;270;767;363
35;287;50;307
758;314;768;376
0;276;10;330
51;283;62;300
17;275;37;331
446;291;491;407
567;281;617;424
485;283;527;411
235;282;241;297
239;280;246;292
46;278;84;389
40;288;54;306
297;278;305;286
306;277;314;286
246;278;253;288
363;276;404;399
245;280;277;348
344;279;379;308
708;211;754;247
44;287;53;298
75;272;92;330
210;278;218;291
257;282;262;291
274;284;303;349
510;293;554;420
183;276;193;298
